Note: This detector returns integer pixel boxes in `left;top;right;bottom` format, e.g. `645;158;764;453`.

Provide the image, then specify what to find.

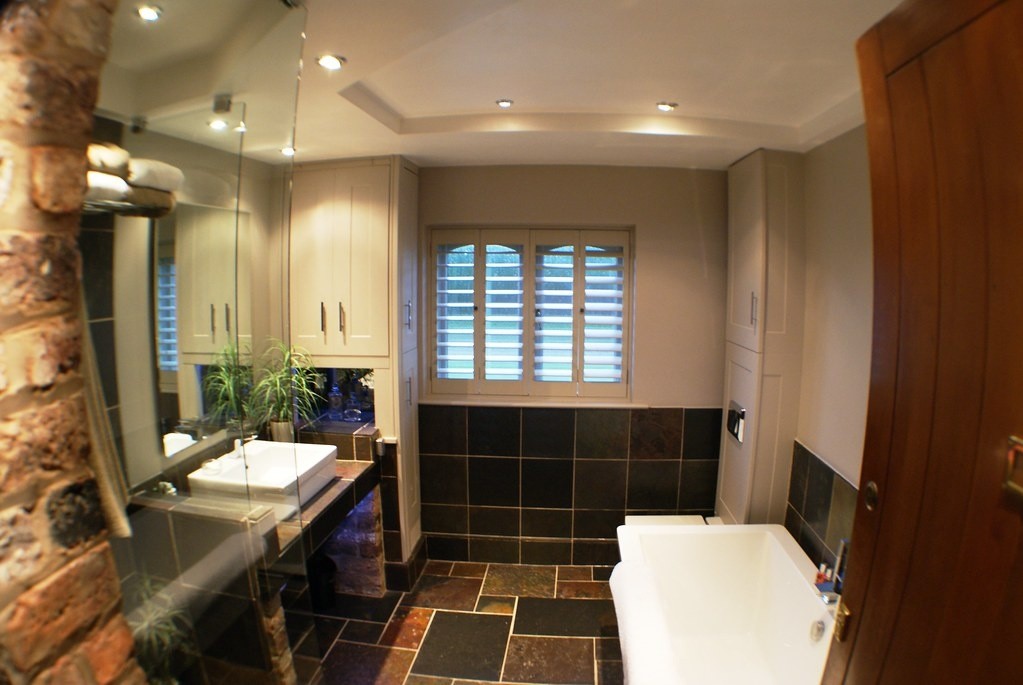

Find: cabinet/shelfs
726;147;803;354
173;206;251;355
716;342;802;526
286;162;425;566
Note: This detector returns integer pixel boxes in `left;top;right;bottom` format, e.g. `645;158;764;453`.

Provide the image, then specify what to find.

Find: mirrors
77;201;252;492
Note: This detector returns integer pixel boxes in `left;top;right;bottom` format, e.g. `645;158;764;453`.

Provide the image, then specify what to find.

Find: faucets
822;540;846;605
233;434;259;461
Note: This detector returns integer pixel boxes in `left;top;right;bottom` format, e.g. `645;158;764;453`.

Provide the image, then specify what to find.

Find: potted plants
246;332;327;444
200;330;253;430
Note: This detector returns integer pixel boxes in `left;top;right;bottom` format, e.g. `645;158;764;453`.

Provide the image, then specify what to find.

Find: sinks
186;440;337;522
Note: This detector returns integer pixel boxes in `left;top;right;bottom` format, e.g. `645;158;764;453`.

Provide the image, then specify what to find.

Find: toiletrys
815;563;834;593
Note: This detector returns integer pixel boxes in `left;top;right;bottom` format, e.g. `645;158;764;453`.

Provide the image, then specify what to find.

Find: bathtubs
608;525;840;685
